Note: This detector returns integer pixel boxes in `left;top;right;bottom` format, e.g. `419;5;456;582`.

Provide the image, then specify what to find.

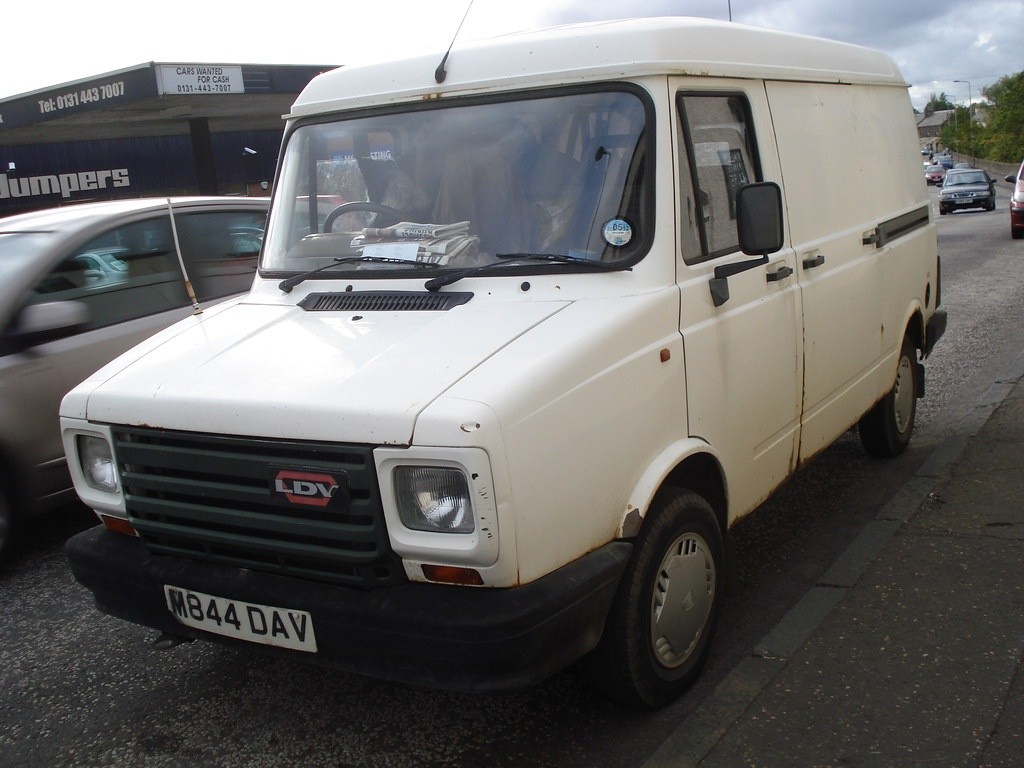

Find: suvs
59;17;949;711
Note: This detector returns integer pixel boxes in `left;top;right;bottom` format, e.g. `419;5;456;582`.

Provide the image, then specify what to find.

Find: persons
40;259;86;302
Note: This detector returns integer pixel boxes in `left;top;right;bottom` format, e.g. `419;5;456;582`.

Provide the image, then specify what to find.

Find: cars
936;169;998;215
1004;159;1024;239
921;147;974;186
0;195;359;564
